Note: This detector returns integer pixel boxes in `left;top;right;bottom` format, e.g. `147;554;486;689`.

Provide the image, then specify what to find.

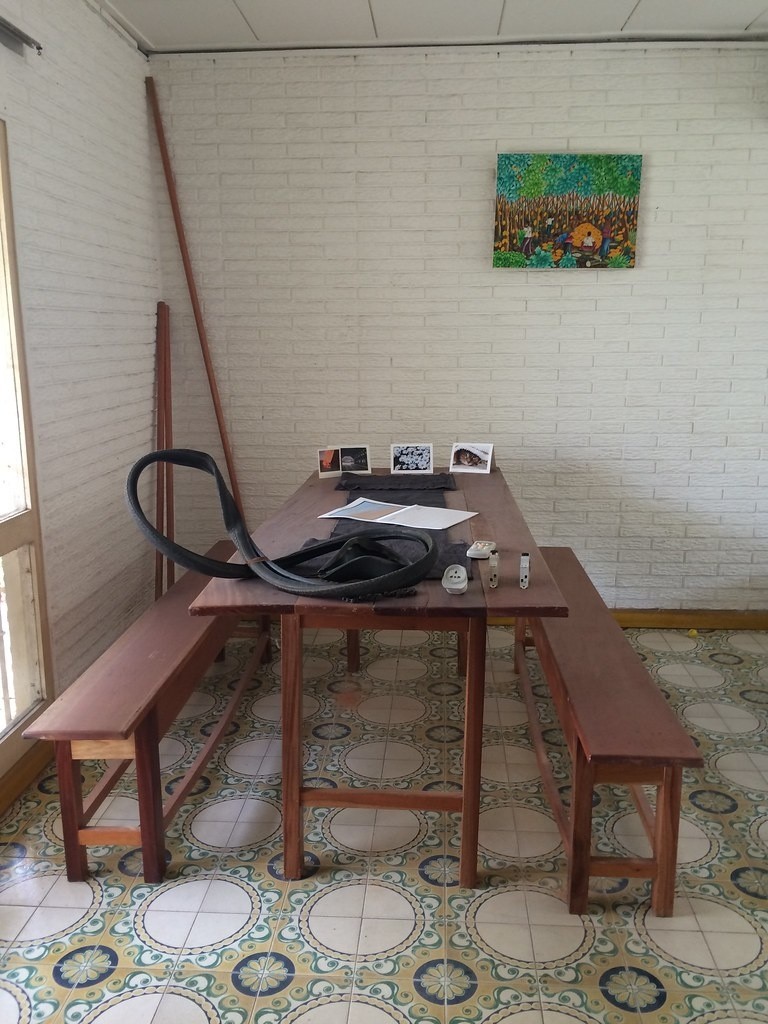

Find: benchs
22;540;272;883
515;546;705;919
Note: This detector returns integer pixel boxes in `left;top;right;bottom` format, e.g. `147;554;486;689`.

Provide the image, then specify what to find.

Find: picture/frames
318;445;371;479
449;442;497;474
391;443;434;474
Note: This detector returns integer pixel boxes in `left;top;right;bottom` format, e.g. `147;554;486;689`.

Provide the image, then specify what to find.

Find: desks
190;467;570;889
